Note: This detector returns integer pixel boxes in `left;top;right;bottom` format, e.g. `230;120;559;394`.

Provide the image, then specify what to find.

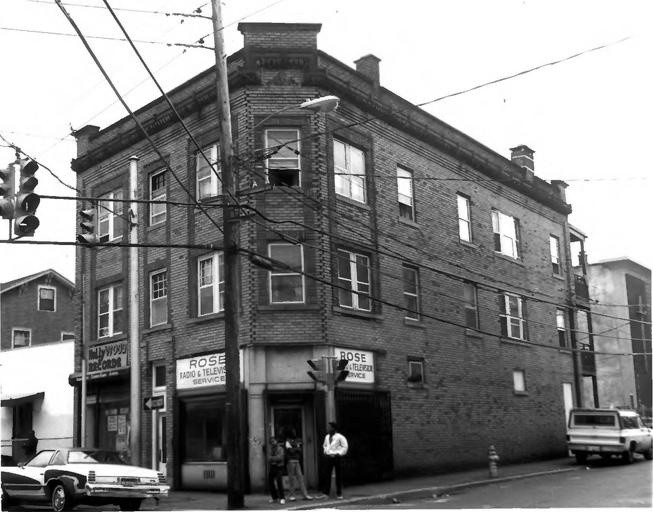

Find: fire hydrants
487;444;500;479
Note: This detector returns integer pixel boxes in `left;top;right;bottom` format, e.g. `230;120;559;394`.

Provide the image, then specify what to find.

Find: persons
285;428;313;501
314;422;348;501
20;429;39;463
266;436;287;506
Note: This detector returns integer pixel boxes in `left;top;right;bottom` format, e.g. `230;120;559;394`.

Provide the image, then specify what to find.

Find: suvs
564;406;652;465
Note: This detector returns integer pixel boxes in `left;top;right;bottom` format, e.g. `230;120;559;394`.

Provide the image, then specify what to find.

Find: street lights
218;92;339;510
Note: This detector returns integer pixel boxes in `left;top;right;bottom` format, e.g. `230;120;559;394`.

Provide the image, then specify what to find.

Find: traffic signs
143;394;164;411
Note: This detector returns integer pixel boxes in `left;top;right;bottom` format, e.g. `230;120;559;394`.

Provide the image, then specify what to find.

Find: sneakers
269;495;343;504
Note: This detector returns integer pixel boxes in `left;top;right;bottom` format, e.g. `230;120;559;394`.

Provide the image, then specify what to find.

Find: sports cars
0;446;172;511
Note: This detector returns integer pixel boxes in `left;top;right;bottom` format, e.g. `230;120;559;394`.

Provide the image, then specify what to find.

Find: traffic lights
328;358;349;384
19;159;38;193
304;358;326;386
0;163;16;220
75;207;96;248
13;191;39;236
